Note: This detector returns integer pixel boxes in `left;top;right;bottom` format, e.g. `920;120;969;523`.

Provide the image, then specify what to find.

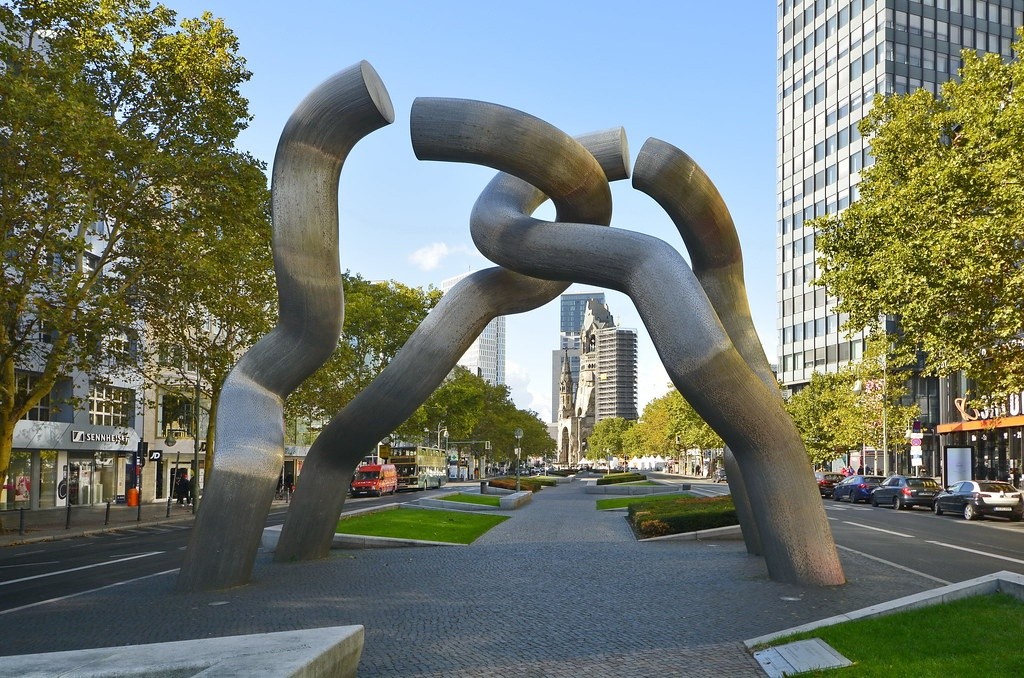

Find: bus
387;446;448;491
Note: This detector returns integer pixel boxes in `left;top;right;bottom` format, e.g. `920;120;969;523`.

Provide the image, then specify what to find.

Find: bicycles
275;489;286;501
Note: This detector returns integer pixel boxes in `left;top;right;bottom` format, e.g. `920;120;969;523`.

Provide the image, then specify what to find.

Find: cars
831;475;887;504
515;465;554;476
933;479;1024;522
814;472;847;498
870;475;942;510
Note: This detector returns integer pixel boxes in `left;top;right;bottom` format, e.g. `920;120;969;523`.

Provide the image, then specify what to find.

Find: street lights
438;423;450;449
852;357;887;477
164;427;200;515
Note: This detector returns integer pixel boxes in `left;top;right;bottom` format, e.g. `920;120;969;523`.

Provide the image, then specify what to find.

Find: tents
577;455;671;469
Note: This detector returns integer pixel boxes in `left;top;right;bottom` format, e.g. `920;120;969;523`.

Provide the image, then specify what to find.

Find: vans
351;464;398;497
448;459;469;482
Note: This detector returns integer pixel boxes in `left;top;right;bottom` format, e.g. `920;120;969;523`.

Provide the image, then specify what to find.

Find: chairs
911;480;920;487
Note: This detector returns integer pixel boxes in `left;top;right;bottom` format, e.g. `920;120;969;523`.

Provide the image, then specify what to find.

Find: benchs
499;490;533;510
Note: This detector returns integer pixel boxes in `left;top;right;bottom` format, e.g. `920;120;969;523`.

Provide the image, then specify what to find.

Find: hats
183;475;187;478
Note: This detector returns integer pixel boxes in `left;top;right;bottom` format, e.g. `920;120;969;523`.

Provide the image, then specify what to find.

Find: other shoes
187;503;193;506
181;504;184;506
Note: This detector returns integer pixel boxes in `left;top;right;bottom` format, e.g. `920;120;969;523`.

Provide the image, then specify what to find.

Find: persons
848;466;856;475
277;472;283;491
857;466;864;475
350;476;356;491
866;467;883;476
841;466;847;473
396;450;405;456
286;472;292;492
189;477;194;499
178;477;193;507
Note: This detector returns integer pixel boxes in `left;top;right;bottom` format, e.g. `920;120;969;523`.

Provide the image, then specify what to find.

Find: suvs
712;468;727;483
